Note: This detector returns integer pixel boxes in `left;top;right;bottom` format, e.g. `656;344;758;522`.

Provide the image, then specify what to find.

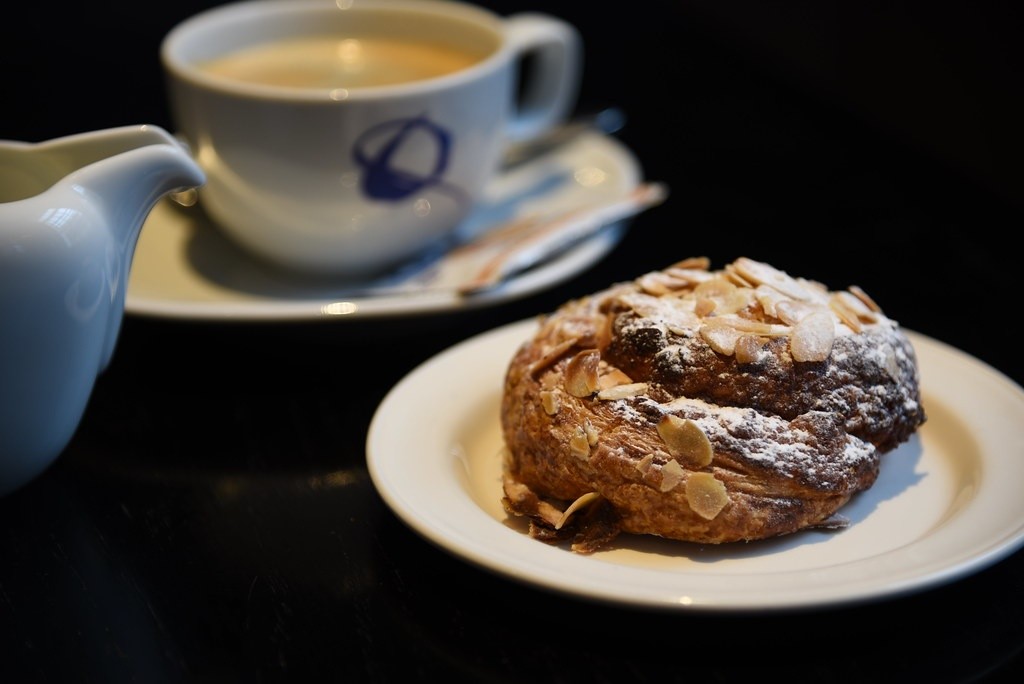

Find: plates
365;315;1023;609
125;122;641;320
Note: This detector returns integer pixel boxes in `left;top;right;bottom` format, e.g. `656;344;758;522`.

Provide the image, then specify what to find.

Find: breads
500;258;928;551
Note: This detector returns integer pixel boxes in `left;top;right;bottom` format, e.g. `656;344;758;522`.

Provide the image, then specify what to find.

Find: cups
159;0;577;276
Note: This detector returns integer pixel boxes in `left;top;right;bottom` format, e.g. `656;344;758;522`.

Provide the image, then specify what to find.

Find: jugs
0;126;205;484
